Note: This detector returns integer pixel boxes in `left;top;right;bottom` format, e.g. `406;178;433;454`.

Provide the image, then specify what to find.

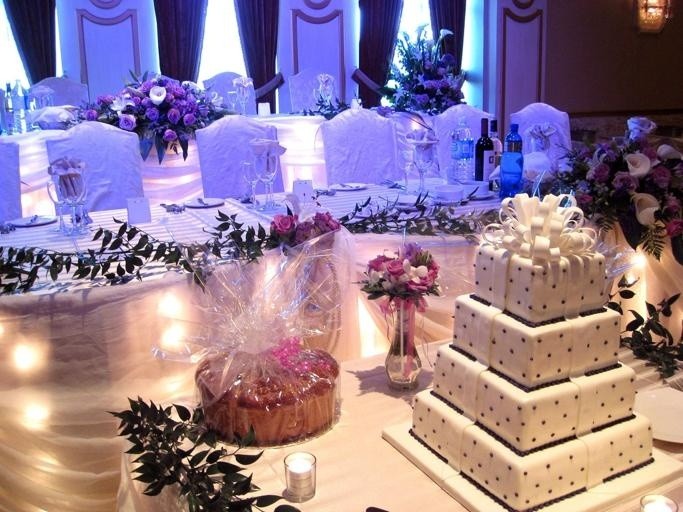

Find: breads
193;346;338;446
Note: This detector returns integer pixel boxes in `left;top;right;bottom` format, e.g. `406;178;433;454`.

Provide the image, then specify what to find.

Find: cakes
410;193;654;512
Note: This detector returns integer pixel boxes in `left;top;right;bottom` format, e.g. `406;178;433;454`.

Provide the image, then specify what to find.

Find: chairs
322;107;398;184
511;103;574;165
45;123;146;214
195;115;285;198
1;71;497;149
0;136;20;219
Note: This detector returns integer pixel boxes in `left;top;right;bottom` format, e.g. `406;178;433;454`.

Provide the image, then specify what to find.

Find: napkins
47;155;84;174
248;137;286;156
525;123;556;149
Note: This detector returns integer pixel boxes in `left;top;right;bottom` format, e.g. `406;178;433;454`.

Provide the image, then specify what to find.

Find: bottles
4;79;30;136
451;117;523;190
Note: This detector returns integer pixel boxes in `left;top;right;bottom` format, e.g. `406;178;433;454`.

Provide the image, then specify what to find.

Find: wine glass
47;173;90;237
228;86;250;116
397;150;433;196
242;155;279;212
315;85;334;108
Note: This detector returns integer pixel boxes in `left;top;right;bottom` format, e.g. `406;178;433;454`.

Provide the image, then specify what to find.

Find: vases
294;258;343;366
378;306;425;392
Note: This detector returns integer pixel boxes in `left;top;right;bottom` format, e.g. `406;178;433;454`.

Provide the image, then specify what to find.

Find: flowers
539;115;681;263
383;21;469;111
362;243;440;318
270;209;340;252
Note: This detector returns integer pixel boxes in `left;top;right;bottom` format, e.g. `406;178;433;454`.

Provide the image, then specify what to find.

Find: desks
121;340;683;512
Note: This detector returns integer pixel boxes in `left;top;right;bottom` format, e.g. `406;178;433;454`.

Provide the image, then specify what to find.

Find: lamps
633;0;675;36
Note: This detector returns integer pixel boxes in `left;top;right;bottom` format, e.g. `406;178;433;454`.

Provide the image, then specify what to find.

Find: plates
433;190;496;204
328;182;367;191
184;198;224;208
5;216;56;228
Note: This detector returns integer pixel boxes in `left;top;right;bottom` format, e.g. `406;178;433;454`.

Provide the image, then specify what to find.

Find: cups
434;180;489;200
641;493;677;512
283;452;318;502
33;96;54;110
